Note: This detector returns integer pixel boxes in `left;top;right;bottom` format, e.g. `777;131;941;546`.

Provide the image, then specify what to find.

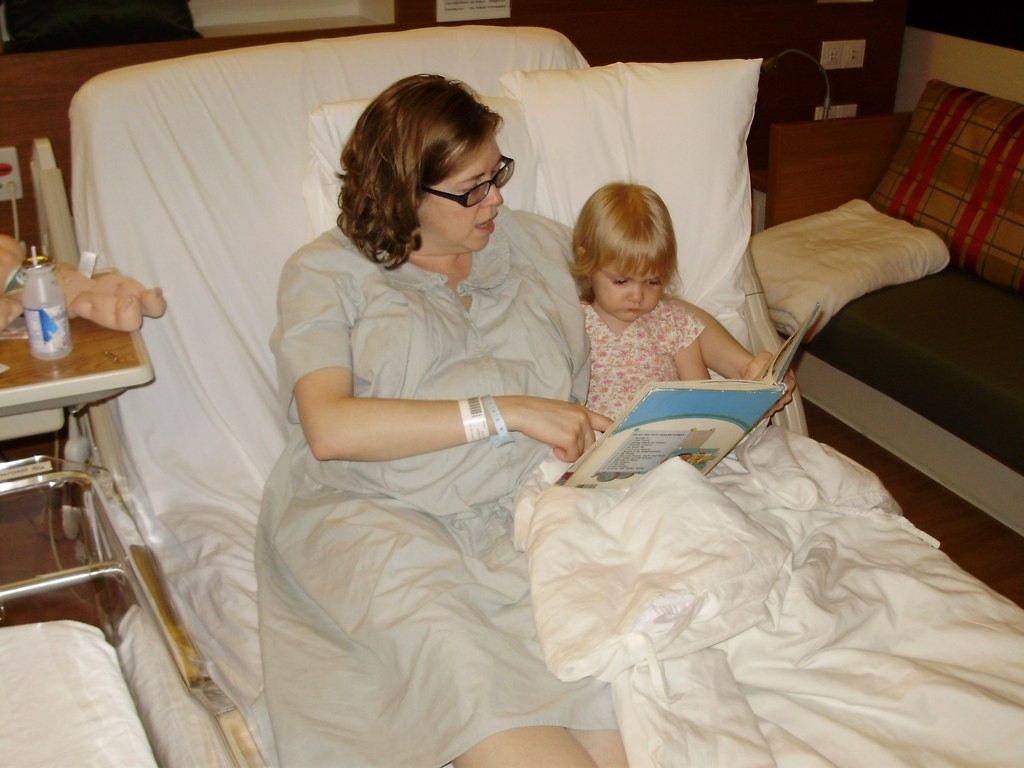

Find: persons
567;182;710;422
252;72;797;768
0;235;167;331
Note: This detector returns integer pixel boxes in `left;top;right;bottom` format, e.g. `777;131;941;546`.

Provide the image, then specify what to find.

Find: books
555;302;821;487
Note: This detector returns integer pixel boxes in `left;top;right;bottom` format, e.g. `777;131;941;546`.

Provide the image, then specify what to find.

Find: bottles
21;255;74;361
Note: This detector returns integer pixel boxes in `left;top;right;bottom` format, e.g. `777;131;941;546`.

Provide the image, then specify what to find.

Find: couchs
770;75;1024;541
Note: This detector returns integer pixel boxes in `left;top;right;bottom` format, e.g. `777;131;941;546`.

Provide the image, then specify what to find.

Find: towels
749;197;951;345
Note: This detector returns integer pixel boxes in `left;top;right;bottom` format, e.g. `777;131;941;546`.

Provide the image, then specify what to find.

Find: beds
30;23;1024;768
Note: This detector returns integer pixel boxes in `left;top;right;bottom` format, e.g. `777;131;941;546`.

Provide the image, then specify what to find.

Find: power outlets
820;39;865;71
0;147;24;203
813;104;857;120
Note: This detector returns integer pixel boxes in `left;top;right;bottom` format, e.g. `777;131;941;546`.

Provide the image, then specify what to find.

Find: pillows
306;92;537;218
499;56;809;434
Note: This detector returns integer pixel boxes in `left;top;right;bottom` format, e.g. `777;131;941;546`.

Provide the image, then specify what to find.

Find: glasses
419;153;515;207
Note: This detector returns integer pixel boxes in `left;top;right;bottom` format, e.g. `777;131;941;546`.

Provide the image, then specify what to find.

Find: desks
0;263;159;453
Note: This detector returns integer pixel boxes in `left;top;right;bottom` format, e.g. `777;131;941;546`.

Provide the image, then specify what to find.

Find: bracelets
482;395;514;449
458;396;489;443
